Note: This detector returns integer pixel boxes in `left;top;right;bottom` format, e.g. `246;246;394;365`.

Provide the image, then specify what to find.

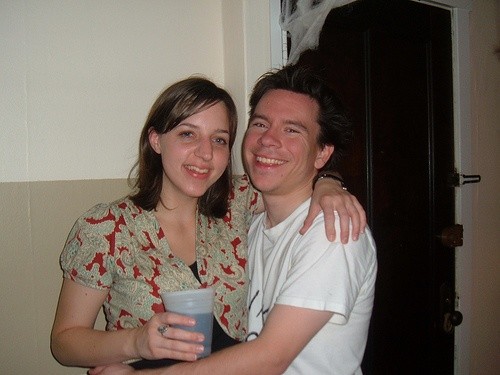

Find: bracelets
311;173;348;190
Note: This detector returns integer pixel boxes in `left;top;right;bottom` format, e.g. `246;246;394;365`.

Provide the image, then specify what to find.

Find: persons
50;73;367;375
86;64;379;375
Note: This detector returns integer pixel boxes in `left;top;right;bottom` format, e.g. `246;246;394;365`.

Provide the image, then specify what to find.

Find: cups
161;288;214;358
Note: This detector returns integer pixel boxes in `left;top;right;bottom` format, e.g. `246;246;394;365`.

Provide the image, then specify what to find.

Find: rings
157;326;168;336
